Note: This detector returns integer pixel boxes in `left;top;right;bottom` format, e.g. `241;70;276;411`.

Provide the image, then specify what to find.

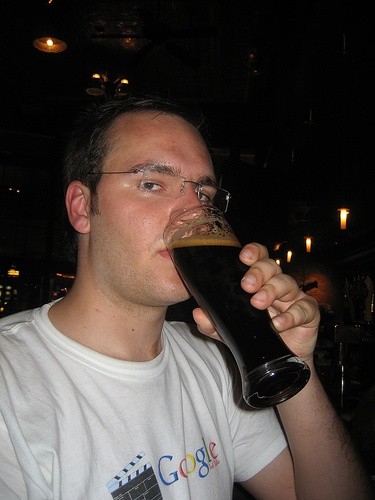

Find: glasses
89;167;232;216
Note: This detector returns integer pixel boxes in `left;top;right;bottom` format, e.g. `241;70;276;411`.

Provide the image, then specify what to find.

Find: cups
163;207;310;409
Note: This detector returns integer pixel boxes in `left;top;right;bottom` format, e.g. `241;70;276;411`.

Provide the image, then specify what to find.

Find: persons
0;93;370;500
